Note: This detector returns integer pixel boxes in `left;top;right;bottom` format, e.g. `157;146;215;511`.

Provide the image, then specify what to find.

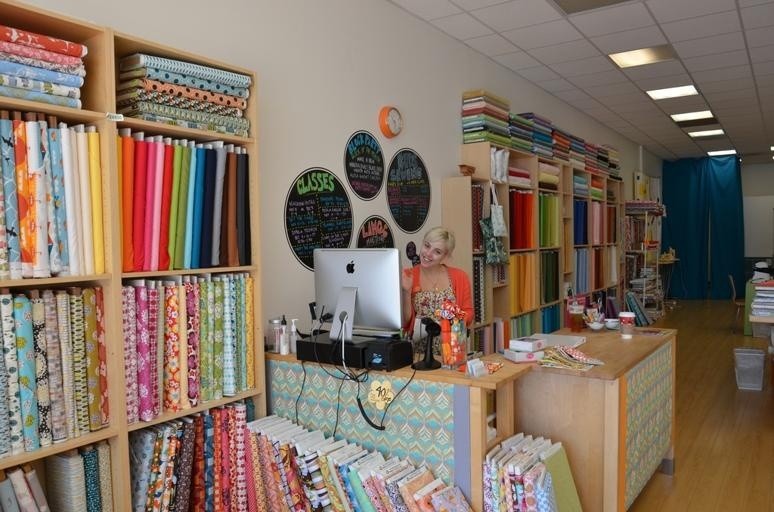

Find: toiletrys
290;318;298;353
280;326;290;356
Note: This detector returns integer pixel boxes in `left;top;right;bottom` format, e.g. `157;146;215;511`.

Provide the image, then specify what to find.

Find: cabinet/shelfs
2;2;267;510
443;142;625;355
627;202;660;312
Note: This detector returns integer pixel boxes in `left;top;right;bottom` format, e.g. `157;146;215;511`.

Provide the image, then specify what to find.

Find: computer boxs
296;332;383;368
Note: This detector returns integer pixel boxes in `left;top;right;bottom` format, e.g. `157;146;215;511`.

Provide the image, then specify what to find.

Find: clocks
378;105;403;139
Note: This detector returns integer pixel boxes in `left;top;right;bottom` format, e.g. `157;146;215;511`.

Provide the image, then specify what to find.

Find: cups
618;311;636;341
268;318;281;354
568;301;584;333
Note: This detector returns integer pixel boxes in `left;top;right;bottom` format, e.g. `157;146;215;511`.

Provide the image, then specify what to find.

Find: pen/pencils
582;313;605;324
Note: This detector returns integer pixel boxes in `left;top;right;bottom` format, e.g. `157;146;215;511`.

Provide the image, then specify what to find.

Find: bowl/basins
603;319;619;331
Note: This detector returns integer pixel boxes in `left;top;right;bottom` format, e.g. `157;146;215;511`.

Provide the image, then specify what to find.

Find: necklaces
422;265;442;292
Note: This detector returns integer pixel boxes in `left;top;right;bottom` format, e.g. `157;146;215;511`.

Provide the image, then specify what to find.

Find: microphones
420;314;441;338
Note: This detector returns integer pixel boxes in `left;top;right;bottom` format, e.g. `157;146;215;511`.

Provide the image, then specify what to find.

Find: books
749;275;774;317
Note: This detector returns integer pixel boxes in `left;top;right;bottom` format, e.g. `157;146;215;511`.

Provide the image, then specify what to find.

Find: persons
400;226;475;356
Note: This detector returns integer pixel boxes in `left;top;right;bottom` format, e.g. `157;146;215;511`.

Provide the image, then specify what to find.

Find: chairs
728;275;744;332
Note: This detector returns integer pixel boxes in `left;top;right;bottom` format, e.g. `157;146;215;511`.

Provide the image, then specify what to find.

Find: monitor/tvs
314;248;405;342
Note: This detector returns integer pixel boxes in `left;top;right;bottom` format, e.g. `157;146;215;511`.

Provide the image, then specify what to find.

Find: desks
750;316;774;354
267;326;678;512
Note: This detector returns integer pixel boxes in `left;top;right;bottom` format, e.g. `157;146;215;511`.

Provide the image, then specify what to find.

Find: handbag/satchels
478;204;510;264
